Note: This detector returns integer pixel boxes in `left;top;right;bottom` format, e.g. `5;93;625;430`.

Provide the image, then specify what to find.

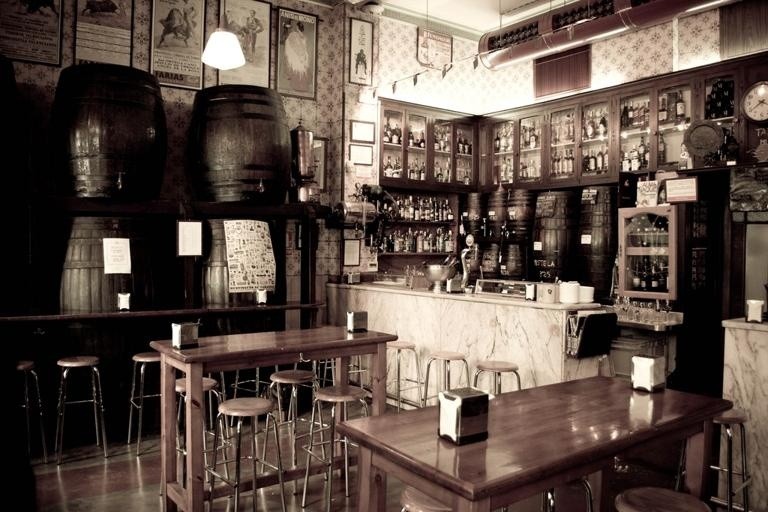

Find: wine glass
422;264;455;292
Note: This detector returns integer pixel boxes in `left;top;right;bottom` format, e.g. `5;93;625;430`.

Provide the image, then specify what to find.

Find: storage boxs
632;354;665;394
171;321;199;350
347;312;368;333
436;388;489;445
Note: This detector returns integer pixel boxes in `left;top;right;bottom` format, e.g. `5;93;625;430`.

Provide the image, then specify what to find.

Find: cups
400;264;416;286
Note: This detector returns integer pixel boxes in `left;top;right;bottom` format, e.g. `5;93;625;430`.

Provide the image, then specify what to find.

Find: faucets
504;230;516;239
255;177;266;193
113;171;127;192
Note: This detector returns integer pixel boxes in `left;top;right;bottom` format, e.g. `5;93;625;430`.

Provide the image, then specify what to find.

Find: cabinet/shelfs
377;58;738;189
616;205;726;300
379;186;459;258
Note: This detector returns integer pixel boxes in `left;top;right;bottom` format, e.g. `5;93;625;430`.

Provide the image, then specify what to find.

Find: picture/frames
314;136;329;192
349;119;375;145
150;1;207;90
342;238;361;268
1;2;63;67
274;6;318;101
350;14;374;87
347;143;373;166
216;1;271;88
74;2;135;65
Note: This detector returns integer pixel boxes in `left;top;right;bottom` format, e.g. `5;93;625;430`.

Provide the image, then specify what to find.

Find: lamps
201;2;246;71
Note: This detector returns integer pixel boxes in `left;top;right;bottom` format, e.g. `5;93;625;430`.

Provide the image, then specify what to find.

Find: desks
337;376;734;512
2;302;325;456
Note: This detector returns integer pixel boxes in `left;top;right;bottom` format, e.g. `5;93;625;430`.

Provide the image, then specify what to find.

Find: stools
616;487;711;511
149;325;398;512
473;361;521;394
56;356;107;463
400;488;454;512
15;359;48;463
422;351;470;408
542;480;593;512
128;352;163;456
714;411;752;512
386;341;421;412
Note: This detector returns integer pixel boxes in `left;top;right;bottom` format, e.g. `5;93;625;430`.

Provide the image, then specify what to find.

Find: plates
559;282;595;304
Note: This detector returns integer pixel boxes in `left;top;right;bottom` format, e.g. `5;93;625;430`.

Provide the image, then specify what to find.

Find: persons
285;22;309;80
245;9;264;56
355;49;366;76
180;0;199;47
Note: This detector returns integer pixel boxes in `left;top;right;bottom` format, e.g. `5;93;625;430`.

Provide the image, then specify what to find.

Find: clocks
738;80;767;122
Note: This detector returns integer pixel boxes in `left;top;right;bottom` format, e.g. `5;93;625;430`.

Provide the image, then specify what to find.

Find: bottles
384;114;472;183
384;193;454;254
491;78;736;183
628;217;668;289
602;295;676;324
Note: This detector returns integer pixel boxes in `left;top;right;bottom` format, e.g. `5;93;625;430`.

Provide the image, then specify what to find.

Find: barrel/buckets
180;220;283;336
575;185;618;298
189;83;296;204
58;215;157;314
464;192;488;237
465;242;526;285
485;191;507;238
52;63;168;202
528;190;578;283
506;188;535;242
